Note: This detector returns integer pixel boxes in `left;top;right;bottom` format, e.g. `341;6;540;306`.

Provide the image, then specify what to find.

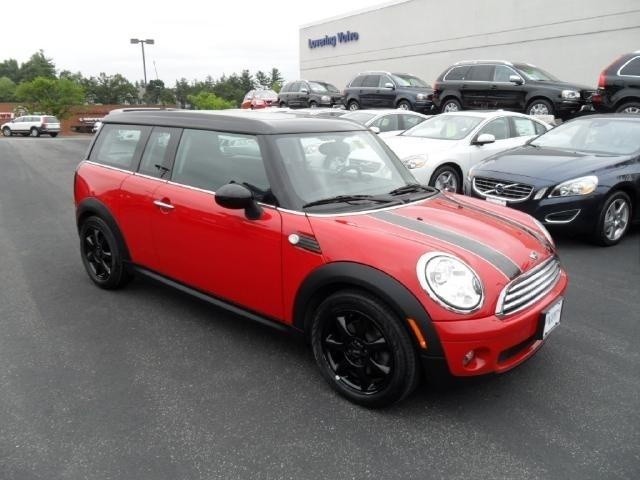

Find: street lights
129;38;156;87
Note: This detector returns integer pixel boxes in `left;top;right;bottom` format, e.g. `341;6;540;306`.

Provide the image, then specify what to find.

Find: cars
344;107;556;203
465;112;640;248
239;87;283;110
0;114;62;138
290;108;431;180
70;96;569;411
592;49;640;116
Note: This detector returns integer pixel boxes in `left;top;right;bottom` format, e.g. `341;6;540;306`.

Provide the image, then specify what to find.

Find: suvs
432;58;600;121
278;79;346;110
340;69;437;115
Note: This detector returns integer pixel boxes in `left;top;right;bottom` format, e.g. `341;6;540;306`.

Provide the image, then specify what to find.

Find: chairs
586;126;640;153
186;132;228;190
